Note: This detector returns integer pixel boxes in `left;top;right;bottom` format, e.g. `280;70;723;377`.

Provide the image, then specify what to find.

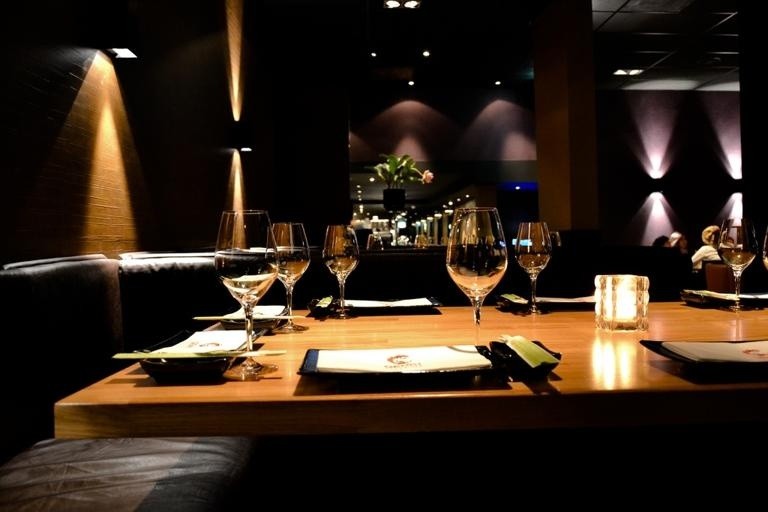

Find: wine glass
264;221;311;335
447;206;508;346
322;223;361;321
717;217;758;313
214;208;281;381
513;220;550;317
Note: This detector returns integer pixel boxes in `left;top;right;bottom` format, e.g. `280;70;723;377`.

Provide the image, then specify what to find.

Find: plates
494;290;596;312
219;304;289;331
637;335;768;370
299;345;507;385
311;295;442;314
680;288;768;308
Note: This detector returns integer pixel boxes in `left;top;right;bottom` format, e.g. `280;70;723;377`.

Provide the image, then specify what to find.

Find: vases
383;190;406;210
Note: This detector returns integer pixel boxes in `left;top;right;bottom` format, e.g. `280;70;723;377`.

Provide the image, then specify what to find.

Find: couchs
0;244;768;512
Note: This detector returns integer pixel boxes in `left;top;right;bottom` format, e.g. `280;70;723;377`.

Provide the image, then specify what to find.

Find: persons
653;225;721;271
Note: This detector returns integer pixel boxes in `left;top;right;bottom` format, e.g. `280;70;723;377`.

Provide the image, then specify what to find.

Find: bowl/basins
139;356;236;383
489;339;564;377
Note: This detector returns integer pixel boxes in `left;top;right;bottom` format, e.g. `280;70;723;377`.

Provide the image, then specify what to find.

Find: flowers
374;152;435;189
